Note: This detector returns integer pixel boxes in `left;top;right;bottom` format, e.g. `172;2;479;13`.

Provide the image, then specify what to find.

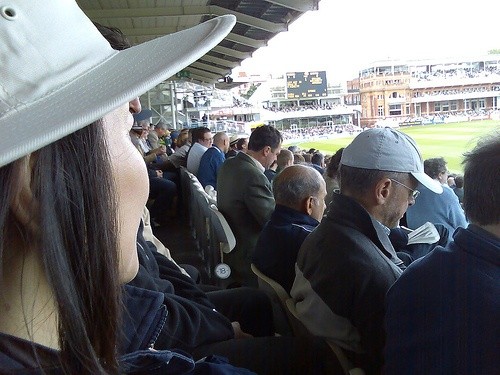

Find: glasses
390;178;420;199
132;129;142;136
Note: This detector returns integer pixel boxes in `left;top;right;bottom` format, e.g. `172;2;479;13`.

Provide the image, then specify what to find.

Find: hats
0;1;237;168
171;131;180;139
164;130;171;137
340;127;443;195
132;121;142;129
229;135;238;146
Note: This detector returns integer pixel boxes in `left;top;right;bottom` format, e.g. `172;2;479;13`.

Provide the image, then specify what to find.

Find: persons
263;102;349;113
290;128;445;375
423;86;500;97
130;107;227;291
257;165;327;293
88;22;343;375
265;144;333;191
277;121;360;141
201;112;245;134
414;66;500;82
388;222;448;268
382;131;500;375
232;96;254;109
407;157;470;259
227;136;249;158
406;109;500;120
322;147;348;211
197;132;231;188
222;125;279;286
1;0;254;375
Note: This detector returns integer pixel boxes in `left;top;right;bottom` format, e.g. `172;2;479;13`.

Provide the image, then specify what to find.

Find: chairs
180;168;363;375
